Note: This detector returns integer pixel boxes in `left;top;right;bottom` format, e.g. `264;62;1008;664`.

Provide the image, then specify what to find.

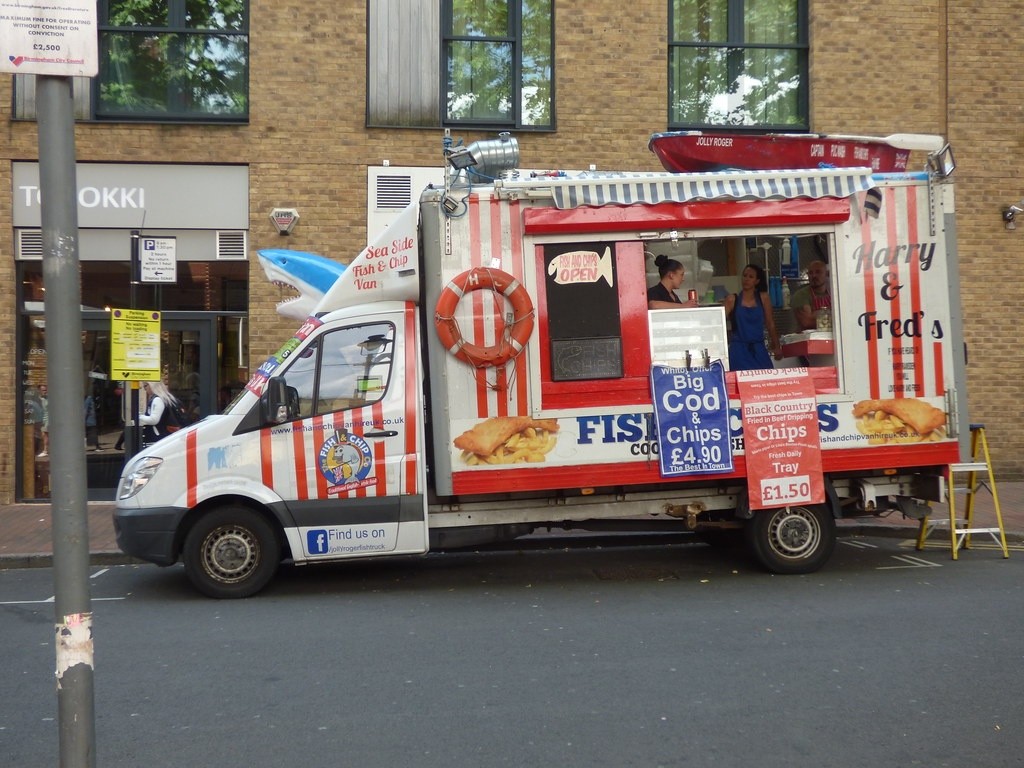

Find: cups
689;290;696;302
706;291;714;304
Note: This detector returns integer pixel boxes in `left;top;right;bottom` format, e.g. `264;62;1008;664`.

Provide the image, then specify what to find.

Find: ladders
915;423;1010;561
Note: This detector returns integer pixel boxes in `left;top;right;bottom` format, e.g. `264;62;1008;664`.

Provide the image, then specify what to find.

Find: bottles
782;275;791;311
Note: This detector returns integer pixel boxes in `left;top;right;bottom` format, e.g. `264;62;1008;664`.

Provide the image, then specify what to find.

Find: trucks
112;129;972;600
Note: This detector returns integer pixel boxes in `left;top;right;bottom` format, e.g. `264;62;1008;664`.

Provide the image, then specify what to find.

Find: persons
648;255;698;308
36;384;49;458
722;265;782;373
114;432;126;450
85;392;103;452
185;373;200;413
792;260;832;329
139;380;185;448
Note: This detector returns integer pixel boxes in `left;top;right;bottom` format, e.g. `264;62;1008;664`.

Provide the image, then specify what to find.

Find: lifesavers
436;268;534;369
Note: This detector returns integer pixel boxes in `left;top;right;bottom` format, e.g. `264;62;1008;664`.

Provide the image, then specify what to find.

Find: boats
648;130;911;172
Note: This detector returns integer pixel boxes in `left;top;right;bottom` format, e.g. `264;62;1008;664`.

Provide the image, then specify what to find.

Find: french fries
460;427;557;465
856;409;947;445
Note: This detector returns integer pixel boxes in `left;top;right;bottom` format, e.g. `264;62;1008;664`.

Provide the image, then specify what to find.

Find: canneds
688;289;697;301
